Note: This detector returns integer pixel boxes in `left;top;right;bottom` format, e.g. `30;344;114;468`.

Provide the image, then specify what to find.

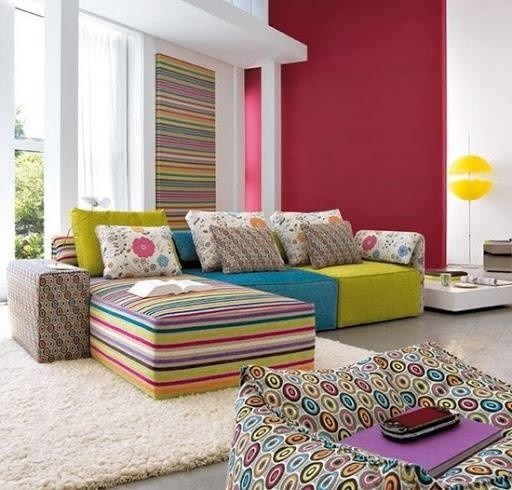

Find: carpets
1;310;383;488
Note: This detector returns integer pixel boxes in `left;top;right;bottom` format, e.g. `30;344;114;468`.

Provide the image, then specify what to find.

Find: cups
439;272;451;287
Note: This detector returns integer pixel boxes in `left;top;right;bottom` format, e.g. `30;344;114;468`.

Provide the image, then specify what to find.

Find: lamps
447;130;495;263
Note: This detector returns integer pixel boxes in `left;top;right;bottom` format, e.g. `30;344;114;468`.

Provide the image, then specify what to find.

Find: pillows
93;218;365;281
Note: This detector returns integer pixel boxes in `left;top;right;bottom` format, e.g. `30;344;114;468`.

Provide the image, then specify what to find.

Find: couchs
4;206;428;402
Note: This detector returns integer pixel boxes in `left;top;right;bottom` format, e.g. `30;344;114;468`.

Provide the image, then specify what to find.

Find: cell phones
379;404;461;440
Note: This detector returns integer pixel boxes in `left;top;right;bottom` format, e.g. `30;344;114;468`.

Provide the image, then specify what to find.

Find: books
424;275;462;281
339;406;503;479
460;275;512;286
128;279;216;299
425;270;467;277
